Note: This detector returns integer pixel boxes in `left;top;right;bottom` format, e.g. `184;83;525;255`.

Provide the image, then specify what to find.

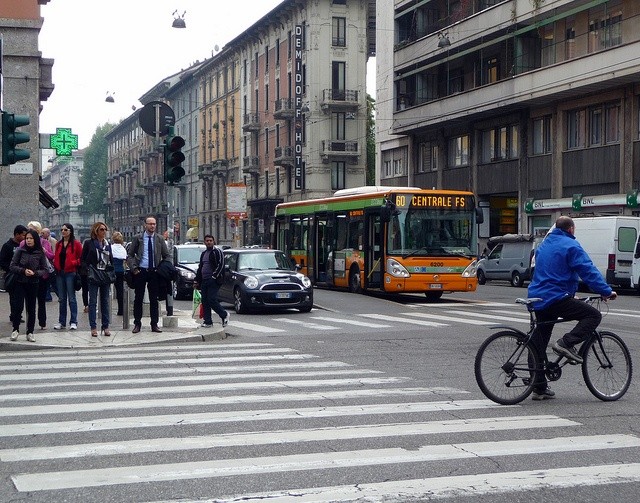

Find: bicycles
474;294;632;404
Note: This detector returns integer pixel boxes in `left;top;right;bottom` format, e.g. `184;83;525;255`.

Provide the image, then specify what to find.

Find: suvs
477;237;545;286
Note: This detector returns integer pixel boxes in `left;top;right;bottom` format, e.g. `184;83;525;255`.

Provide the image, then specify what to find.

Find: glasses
146;223;156;225
100;228;107;230
61;229;70;231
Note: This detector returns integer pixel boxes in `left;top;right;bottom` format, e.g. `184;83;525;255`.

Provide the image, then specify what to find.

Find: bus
274;187;483;299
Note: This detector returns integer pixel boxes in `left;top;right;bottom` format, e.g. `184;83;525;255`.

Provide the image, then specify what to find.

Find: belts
139;268;155;272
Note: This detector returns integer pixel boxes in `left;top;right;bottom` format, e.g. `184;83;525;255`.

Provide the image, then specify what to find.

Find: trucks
630;228;640;295
530;216;640;293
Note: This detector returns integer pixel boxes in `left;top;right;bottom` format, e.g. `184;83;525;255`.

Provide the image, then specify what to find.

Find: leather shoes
132;325;140;333
152;327;162;332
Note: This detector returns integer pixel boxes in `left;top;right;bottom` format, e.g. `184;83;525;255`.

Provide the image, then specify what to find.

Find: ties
148;235;153;269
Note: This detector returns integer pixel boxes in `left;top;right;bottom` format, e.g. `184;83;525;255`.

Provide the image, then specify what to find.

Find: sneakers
54;322;66;329
10;330;19;341
20;320;25;323
552;337;584;363
222;310;230;326
27;333;35;341
39;320;46;329
197;323;213;329
69;322;77;330
532;385;555;400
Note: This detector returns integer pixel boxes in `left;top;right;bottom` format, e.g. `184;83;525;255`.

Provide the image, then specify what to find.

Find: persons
192;235;230;327
9;230;47;342
81;220;115;336
110;230;128;317
19;221;56;330
41;227;61;302
1;224;28;322
79;251;89;313
527;215;617;400
127;216;172;333
54;222;83;330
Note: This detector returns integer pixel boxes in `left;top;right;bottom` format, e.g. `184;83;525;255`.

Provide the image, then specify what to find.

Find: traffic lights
2;112;29;165
165;137;186;183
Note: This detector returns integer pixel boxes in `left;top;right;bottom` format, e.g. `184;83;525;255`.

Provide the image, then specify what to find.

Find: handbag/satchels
74;258;82;291
86;263;117;285
192;287;204;318
5;272;18;292
126;270;138;288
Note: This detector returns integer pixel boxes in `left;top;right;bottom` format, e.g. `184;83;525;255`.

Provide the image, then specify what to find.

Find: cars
218;249;313;314
166;245;219;300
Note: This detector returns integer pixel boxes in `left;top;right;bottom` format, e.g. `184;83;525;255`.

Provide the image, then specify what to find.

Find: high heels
84;304;89;312
101;325;111;335
91;328;98;336
